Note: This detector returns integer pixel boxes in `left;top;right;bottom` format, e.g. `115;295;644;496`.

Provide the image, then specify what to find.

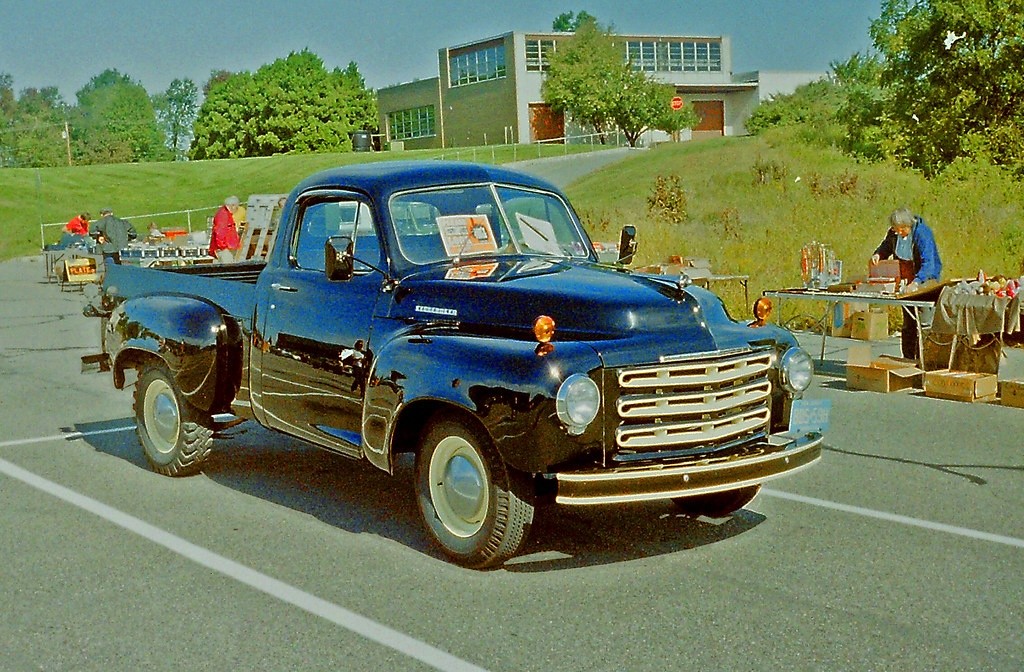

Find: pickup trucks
78;158;826;571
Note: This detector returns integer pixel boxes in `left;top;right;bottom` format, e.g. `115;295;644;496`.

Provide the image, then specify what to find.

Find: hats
100;207;112;213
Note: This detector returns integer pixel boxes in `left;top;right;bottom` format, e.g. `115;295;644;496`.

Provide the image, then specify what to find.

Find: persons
869;208;942;368
92;208;137;272
61;211;91;236
278;197;287;208
209;195;242;263
146;222;161;236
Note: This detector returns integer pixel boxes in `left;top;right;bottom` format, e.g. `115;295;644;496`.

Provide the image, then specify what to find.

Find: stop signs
670;97;683;111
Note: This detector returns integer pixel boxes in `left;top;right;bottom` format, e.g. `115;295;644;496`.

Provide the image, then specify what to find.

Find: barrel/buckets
354;131;372;151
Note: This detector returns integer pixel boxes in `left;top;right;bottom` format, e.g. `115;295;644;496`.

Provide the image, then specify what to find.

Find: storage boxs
922;369;999;403
850;311;890;342
647;262;680;275
1000;378;1024;408
834;354;926;392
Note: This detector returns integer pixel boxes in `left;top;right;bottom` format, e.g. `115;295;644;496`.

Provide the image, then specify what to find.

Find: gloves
903;282;919;293
869;253;880;266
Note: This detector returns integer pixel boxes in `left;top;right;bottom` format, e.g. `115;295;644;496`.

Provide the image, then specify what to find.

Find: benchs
306;247;442;272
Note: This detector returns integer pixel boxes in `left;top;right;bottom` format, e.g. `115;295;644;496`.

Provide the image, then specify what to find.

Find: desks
41;247;213;291
703;275;749;319
762;290;934;372
